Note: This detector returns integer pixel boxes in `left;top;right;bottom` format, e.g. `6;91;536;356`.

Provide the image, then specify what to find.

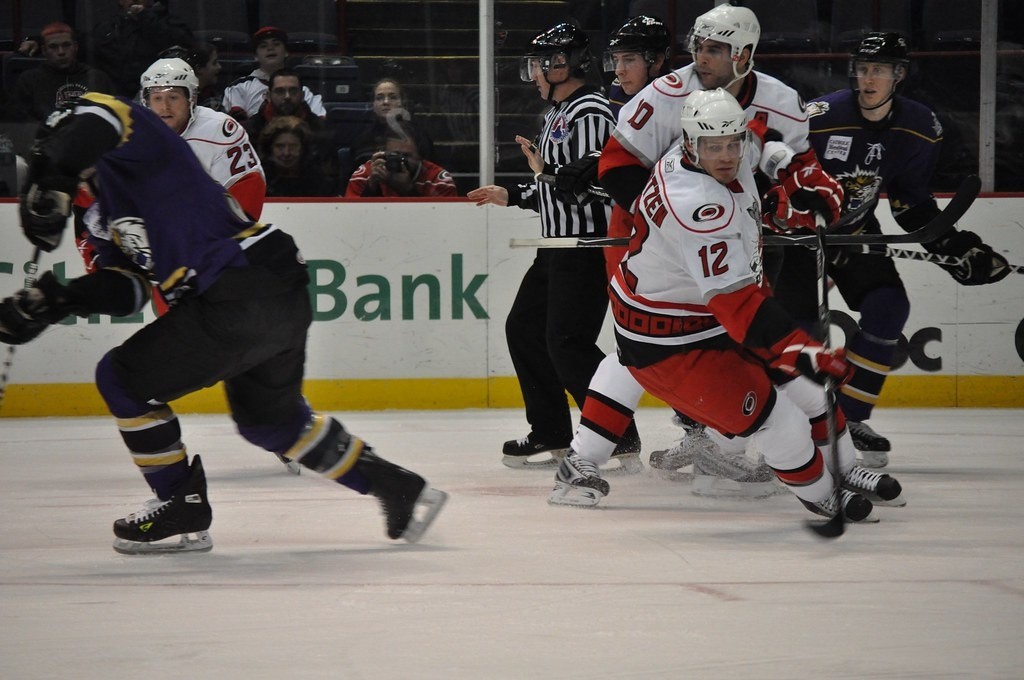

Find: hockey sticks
0;244;43;402
800;212;847;539
509;172;985;250
843;244;1024;274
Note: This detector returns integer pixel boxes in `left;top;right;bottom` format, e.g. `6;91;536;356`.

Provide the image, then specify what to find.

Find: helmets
689;2;761;78
607;14;672;61
850;31;910;65
681;86;749;151
523;23;592;59
140;57;200;104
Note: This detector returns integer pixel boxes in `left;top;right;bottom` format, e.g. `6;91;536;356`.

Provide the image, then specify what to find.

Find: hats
254;26;290;45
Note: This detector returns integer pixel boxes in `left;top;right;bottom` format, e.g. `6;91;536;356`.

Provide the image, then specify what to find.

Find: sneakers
548;447;610;506
598;436;644;479
502;430;575;470
691;449;777;498
369;456;447;544
649;428;715;484
847;421;890;468
839;464;906;507
796;473;881;523
113;454;212;555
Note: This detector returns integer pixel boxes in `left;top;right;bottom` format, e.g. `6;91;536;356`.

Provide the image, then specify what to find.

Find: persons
467;0;1004;538
3;89;449;556
1;1;460;197
73;54;300;476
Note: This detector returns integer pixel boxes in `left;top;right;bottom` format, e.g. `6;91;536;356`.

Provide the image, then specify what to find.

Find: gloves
0;270;69;344
777;147;844;230
763;184;803;234
939;230;1010;286
19;160;79;253
770;330;855;389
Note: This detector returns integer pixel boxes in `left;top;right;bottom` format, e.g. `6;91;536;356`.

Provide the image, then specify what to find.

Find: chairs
0;0;390;198
574;0;1024;193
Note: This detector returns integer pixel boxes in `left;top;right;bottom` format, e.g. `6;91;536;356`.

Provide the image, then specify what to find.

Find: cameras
380;151;408;173
